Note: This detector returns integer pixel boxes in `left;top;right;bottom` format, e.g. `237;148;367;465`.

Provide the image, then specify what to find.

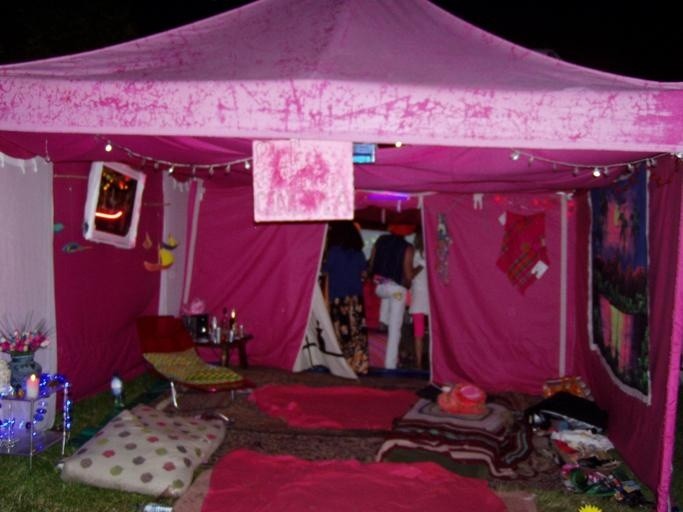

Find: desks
0;381;73;469
193;332;254;369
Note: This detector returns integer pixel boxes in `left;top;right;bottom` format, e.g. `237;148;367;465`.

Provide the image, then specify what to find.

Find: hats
437;382;487;414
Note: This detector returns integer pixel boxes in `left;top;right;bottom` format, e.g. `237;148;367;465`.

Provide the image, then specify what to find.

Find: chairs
137;314;258;408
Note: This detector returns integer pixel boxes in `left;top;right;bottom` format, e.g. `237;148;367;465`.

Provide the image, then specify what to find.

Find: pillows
54;405;227;498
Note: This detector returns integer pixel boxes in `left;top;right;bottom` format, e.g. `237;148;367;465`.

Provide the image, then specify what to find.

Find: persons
370;222;430;369
326;220;369;375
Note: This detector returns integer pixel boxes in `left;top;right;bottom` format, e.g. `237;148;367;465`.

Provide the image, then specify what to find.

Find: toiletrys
210;306;243;344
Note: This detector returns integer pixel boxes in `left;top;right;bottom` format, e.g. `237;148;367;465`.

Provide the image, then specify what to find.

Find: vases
7;350;43;385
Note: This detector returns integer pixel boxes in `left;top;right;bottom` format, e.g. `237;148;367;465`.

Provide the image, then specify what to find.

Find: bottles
110;373;124;408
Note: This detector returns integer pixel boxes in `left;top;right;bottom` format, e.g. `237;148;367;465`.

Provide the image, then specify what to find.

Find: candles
25;373;40;399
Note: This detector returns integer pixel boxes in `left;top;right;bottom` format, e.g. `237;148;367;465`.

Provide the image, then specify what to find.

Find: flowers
0;310;52;357
